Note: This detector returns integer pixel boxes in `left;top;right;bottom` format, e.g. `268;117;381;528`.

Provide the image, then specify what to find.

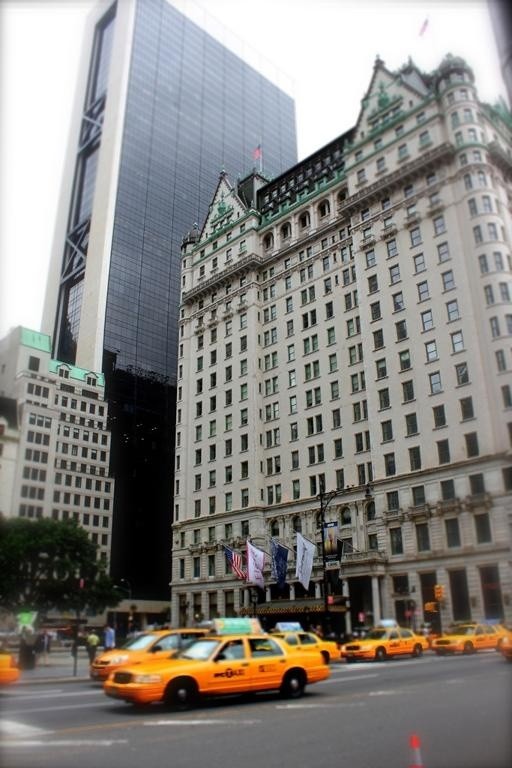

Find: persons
310;624;324;639
0;624;116;667
418;624;433;635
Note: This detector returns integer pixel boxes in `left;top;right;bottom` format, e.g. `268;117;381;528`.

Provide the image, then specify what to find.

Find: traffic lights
434;585;442;599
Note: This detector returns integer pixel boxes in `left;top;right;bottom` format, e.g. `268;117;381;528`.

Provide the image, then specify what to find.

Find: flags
254;144;261;160
225;548;247;579
295;532;316;592
322;527;344;585
271;537;288;589
246;539;265;590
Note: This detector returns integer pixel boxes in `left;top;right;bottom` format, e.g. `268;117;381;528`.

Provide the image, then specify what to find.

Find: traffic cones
409;737;422;766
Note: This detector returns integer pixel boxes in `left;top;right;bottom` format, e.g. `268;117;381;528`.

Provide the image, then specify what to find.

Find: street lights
317;481;335;627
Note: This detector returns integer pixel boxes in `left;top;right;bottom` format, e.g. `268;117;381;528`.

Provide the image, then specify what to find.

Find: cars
431;622;512;661
90;629;341;712
0;654;18;685
340;627;428;661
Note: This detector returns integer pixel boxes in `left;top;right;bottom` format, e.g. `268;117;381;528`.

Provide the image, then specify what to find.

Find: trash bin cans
19;645;37;670
